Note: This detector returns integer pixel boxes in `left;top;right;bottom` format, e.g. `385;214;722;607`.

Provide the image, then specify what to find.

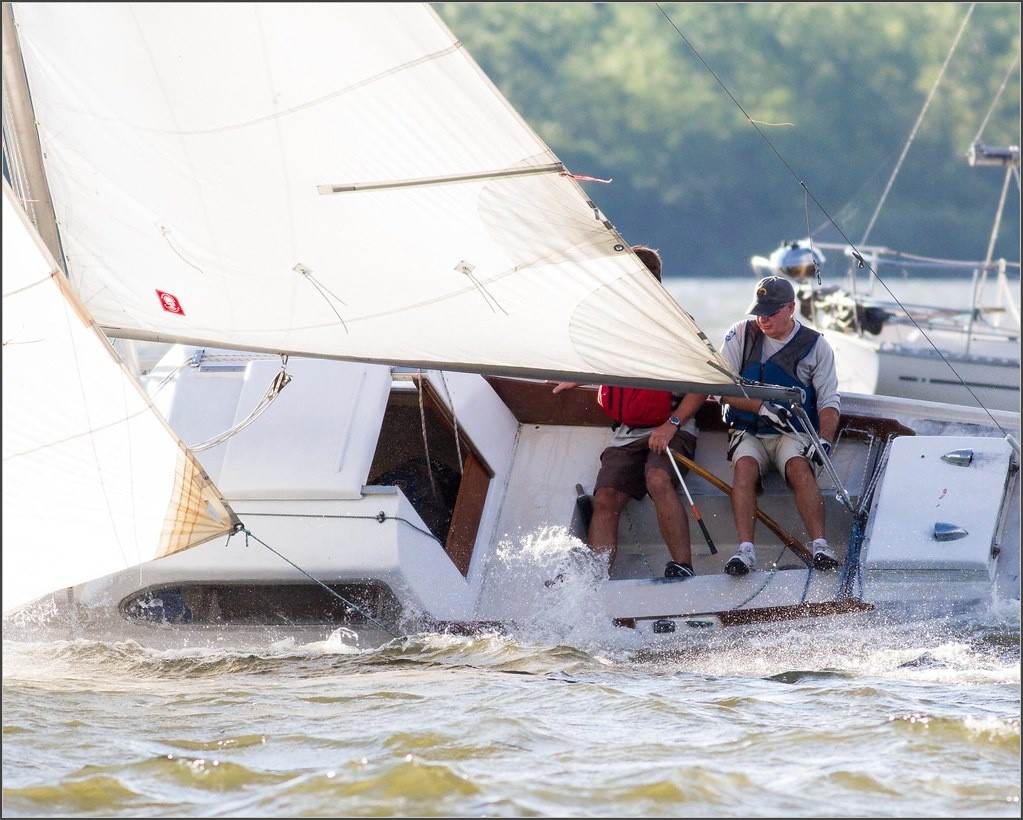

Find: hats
749;276;794;316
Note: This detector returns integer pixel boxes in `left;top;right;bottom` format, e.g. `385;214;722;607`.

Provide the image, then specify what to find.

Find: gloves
799;436;832;466
756;401;793;428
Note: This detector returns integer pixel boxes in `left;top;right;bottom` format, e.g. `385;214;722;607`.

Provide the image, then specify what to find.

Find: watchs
669;415;682;431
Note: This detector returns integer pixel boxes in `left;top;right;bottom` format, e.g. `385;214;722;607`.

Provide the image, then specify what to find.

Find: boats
748;2;1021;413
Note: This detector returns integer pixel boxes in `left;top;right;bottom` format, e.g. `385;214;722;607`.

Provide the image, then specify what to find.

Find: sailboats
1;0;1023;644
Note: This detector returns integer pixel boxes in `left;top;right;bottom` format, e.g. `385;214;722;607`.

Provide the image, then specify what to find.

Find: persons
711;276;842;576
784;262;818;323
542;246;710;581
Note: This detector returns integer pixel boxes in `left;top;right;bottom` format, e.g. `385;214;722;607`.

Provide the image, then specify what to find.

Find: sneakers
663;560;696;577
813;542;840;568
724;546;757;576
